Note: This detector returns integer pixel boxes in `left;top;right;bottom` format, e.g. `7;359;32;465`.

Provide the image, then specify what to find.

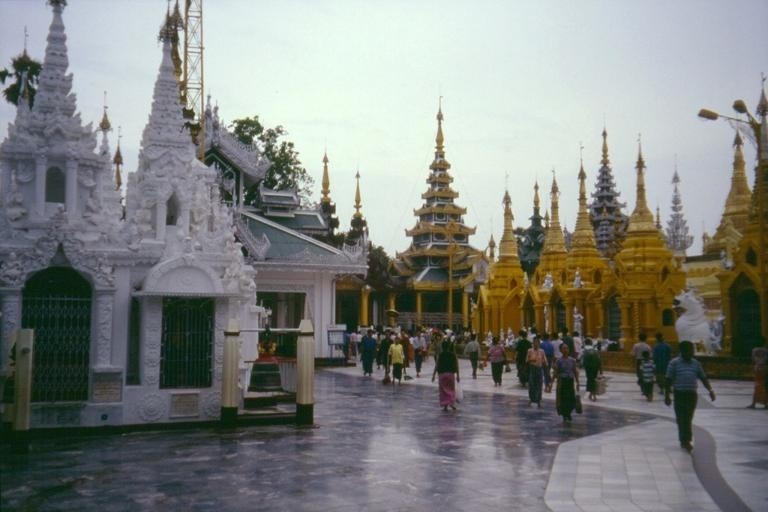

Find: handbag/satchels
575;390;584;414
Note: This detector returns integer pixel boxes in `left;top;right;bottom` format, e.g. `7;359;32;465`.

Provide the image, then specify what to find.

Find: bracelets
709;388;714;391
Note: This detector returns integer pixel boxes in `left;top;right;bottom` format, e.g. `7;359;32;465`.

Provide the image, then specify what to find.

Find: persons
746;335;768;409
663;341;716;452
